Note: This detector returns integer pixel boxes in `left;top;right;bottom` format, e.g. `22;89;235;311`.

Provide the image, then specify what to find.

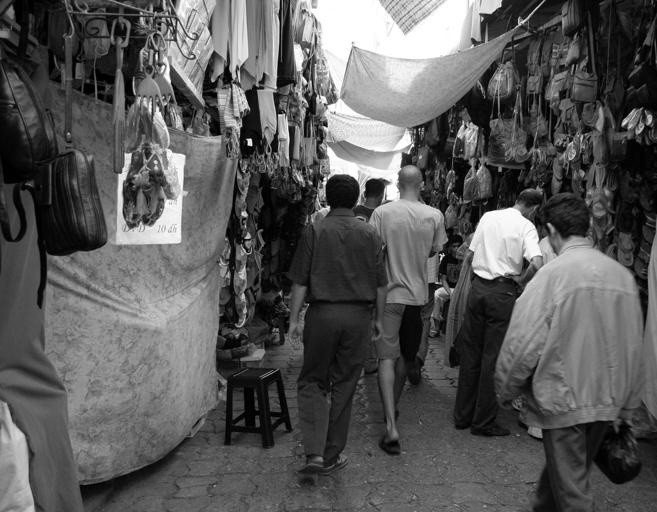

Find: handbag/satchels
0;53;107;255
446;0;595;229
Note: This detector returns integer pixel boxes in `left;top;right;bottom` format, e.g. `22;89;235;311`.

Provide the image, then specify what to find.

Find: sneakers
303;455;349;474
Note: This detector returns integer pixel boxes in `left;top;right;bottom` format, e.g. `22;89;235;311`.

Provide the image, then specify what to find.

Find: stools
224;366;293;449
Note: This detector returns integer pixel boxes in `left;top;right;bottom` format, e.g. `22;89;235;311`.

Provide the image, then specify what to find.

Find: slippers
379;438;402;454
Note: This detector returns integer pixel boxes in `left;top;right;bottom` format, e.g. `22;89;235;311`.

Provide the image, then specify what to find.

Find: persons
452;189;656;511
286;165;464;478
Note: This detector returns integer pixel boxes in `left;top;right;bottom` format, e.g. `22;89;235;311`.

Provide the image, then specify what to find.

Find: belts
499;276;513;285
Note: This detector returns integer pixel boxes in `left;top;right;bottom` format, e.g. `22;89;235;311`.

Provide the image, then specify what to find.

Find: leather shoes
456;418;511;437
